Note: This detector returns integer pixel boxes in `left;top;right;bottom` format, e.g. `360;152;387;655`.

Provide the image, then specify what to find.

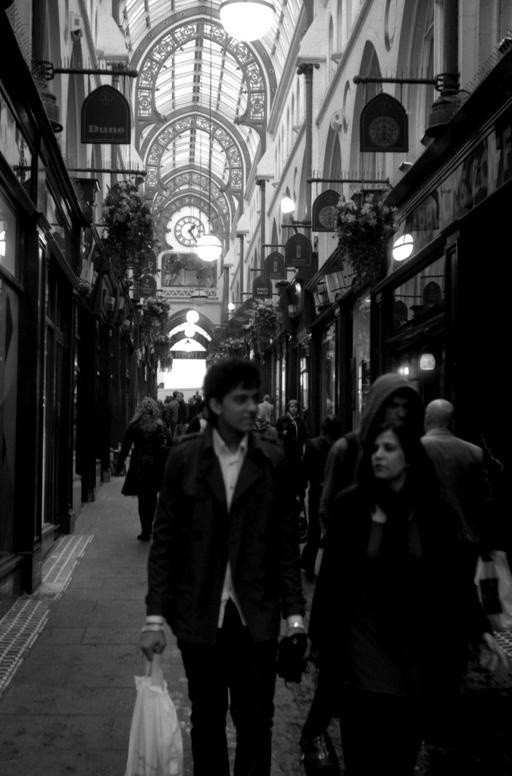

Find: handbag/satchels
126;677;184;776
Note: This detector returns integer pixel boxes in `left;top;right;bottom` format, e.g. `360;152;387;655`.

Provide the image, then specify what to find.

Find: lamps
184;288;209;339
196;33;222;263
219;0;275;43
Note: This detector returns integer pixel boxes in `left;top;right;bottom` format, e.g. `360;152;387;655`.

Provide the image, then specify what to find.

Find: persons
112;359;306;776
299;374;512;776
